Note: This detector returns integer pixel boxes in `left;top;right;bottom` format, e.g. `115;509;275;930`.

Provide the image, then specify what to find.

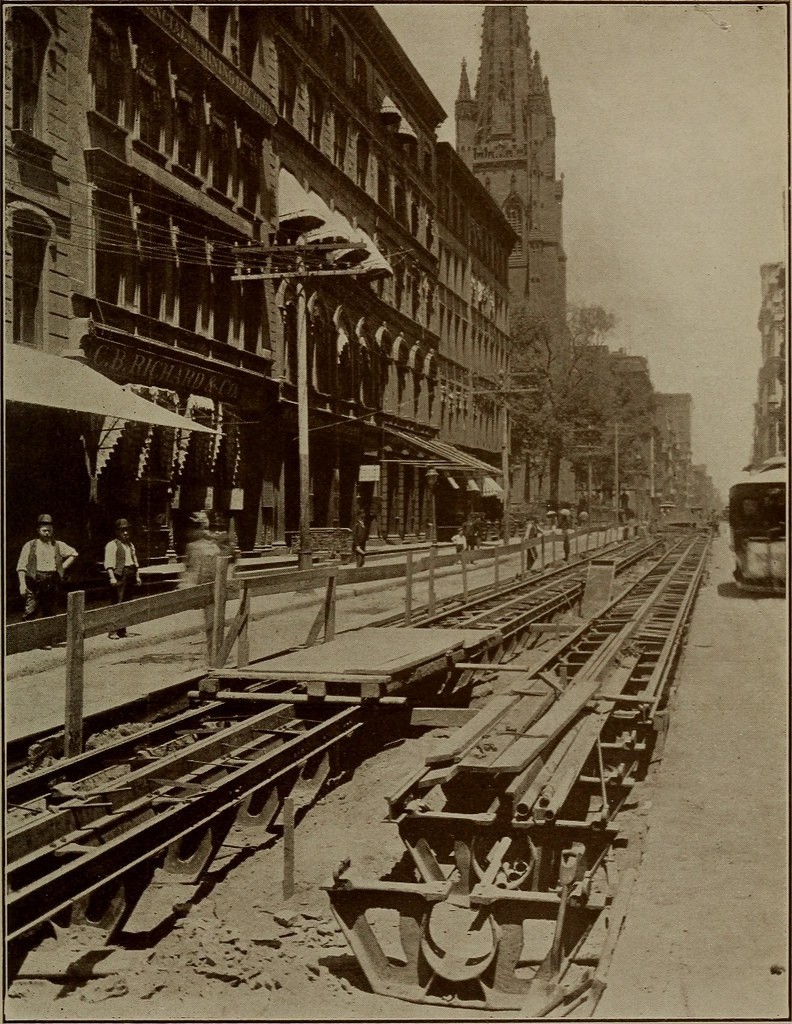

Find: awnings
381;425;506;496
1;341;227;436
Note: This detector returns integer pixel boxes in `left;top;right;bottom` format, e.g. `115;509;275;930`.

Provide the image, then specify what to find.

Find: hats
115;519;132;529
38;515;53;522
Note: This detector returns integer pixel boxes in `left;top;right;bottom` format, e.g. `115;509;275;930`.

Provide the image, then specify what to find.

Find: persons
558;514;569;561
15;513;80;650
523;516;545;569
449;514;480;565
352;510;370;568
546;506;589;527
104;517;142;640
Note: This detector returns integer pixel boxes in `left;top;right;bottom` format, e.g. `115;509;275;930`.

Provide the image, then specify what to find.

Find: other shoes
109;631;120;639
40;644;51;650
